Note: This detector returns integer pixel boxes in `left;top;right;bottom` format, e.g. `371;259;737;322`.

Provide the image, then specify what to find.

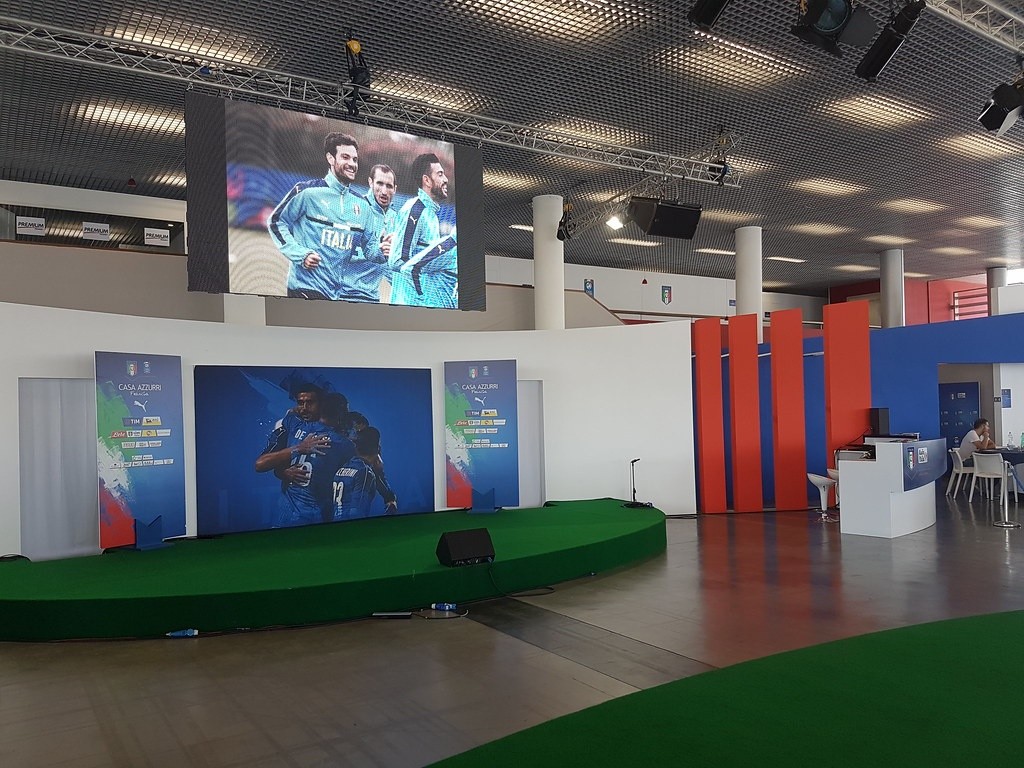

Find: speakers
435;528;495;567
627;196;703;240
869;408;890;437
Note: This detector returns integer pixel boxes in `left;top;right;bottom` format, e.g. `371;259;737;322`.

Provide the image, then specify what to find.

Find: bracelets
290;446;299;459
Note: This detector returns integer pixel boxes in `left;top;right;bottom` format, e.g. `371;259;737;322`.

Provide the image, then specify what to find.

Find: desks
974;448;1024;501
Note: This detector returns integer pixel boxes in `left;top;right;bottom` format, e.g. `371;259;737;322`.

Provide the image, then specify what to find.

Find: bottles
954;436;960;448
1008;432;1014;451
1021;433;1024;452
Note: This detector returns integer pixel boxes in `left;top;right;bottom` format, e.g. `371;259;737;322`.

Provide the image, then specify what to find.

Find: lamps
789;0;856;57
128;175;136;189
556;211;578;241
686;0;730;30
707;159;727;181
977;76;1024;137
601;187;702;240
855;0;928;83
436;528;496;567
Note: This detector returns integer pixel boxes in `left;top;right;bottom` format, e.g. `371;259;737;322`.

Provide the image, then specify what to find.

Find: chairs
945;447;1018;506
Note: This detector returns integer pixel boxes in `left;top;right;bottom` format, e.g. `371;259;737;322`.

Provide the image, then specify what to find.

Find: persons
255;384;399;527
266;132;393;300
339;164;397;304
389;153;449;304
959;418;995;491
397;225;458;310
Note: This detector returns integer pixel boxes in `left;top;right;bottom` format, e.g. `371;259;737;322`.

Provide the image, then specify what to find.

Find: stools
806;468;839;524
